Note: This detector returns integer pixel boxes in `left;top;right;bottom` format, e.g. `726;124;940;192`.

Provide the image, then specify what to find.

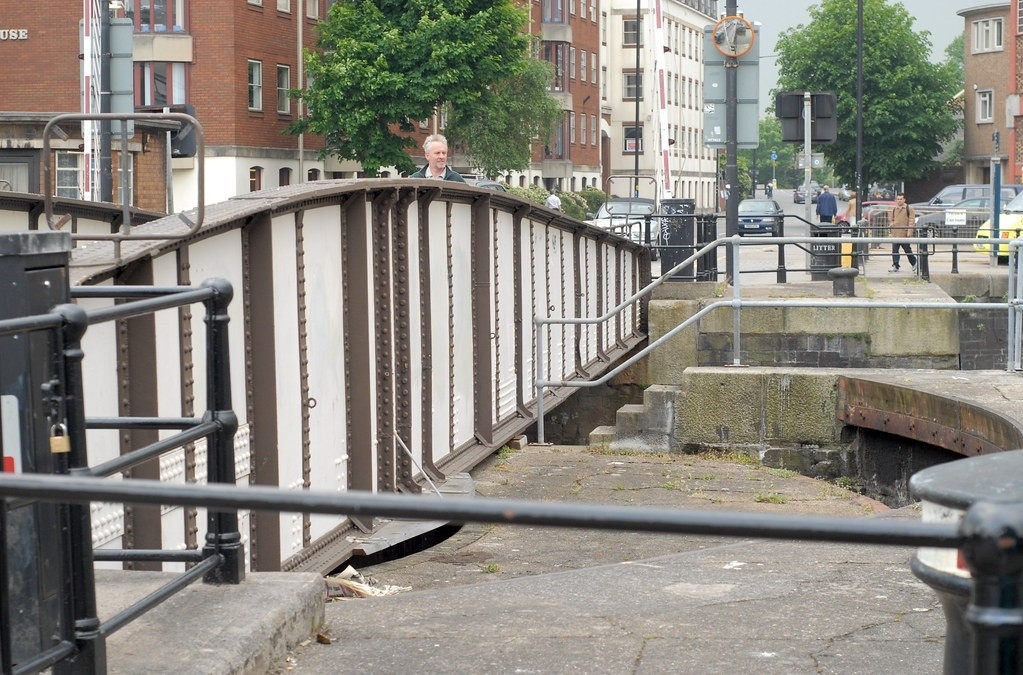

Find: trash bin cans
809;222;842;282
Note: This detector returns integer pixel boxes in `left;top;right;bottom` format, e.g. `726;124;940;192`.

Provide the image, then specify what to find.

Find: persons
408;134;465;183
888;192;920;271
545;189;562;213
766;180;774;199
816;185;891;230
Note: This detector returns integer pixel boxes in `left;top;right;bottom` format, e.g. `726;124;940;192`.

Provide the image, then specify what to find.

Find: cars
586;196;661;262
803;181;821;195
793;185;819;204
738;199;784;238
973;188;1023;265
834;183;1023;245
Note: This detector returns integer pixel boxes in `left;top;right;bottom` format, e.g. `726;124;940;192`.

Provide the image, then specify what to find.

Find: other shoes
913;259;917;271
888;267;899;272
